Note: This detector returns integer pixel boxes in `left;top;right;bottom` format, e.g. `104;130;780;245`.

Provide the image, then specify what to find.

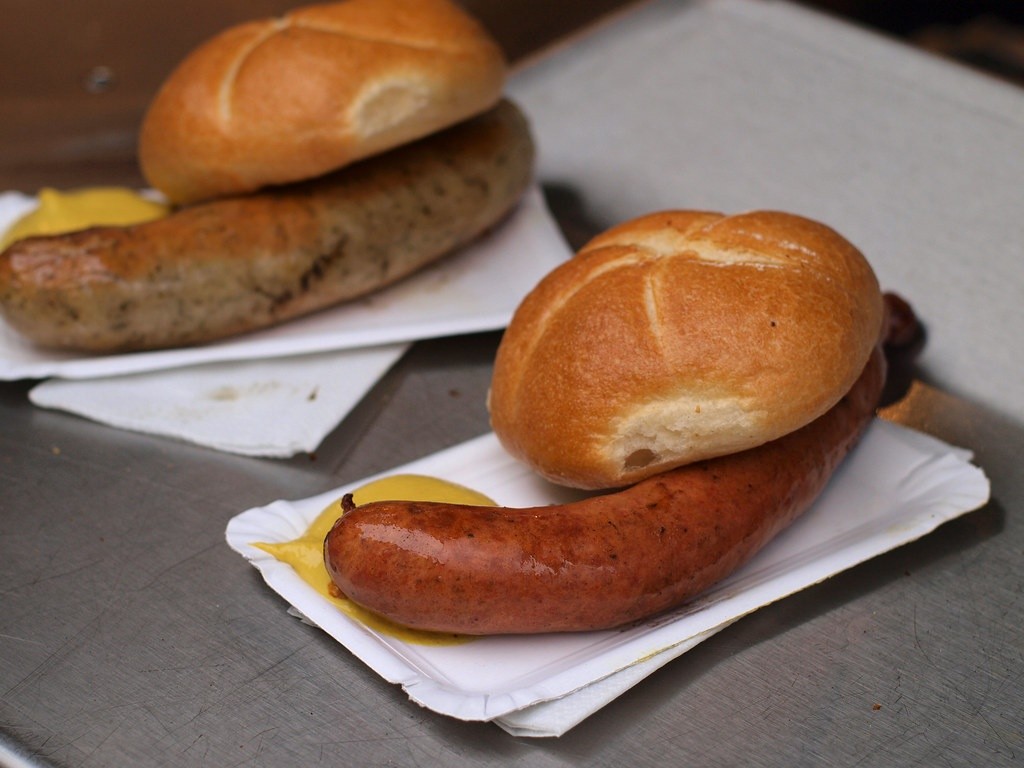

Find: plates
222;403;992;724
0;173;577;383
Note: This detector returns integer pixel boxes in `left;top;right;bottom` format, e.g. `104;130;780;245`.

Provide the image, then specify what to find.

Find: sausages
325;289;887;631
0;94;538;351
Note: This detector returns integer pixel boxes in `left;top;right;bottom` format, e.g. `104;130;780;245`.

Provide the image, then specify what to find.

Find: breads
490;208;883;488
140;0;504;201
